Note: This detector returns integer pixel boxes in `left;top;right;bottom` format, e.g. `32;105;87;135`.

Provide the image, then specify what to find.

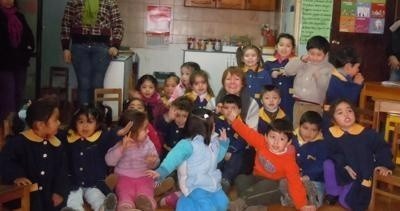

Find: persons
60;0;124;106
1;0;34;119
0;33;399;210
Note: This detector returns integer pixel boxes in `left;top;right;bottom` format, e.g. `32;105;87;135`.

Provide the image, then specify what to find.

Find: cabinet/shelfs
184;0;276;11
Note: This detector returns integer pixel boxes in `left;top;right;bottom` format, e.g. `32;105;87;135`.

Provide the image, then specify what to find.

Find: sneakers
222;176;232;188
227;198;248;211
99;177;181;211
246;206;269;211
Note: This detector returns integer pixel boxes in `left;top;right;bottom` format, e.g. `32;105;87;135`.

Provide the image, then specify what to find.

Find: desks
0;182;39;211
358;79;400;124
183;50;277;97
52;49;134;122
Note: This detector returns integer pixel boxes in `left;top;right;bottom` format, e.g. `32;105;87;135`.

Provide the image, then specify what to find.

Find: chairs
48;65;69;104
93;88;123;126
3;112;15;139
366;99;400;211
